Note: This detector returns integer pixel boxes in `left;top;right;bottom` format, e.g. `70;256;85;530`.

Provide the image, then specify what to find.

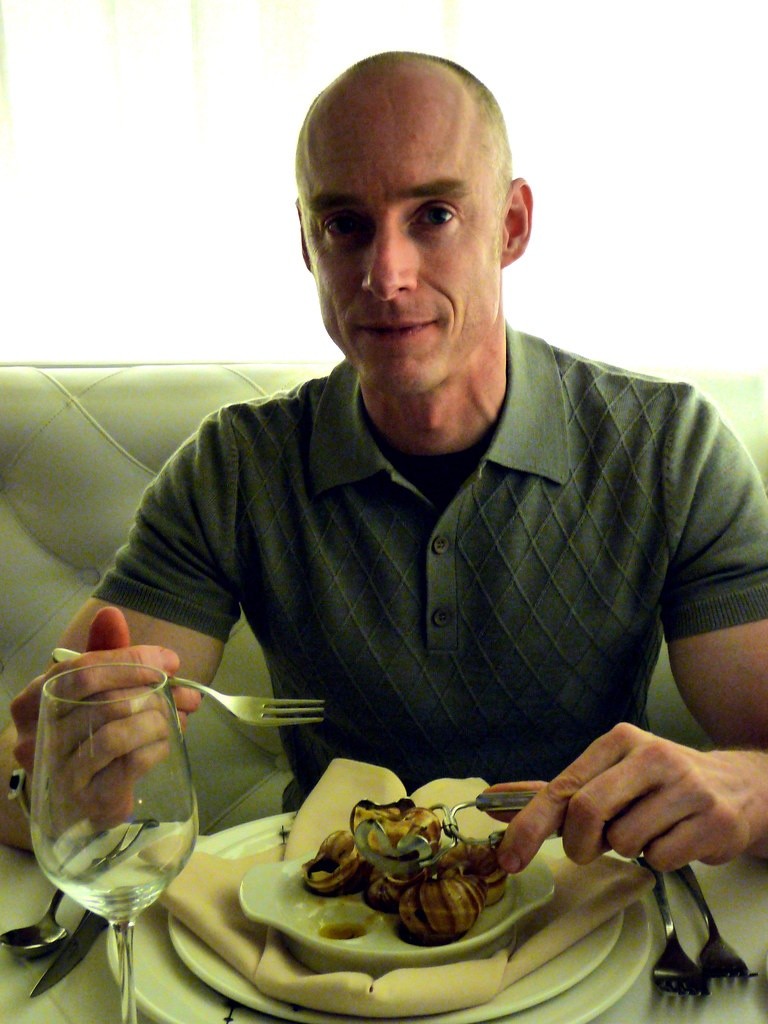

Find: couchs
1;363;768;842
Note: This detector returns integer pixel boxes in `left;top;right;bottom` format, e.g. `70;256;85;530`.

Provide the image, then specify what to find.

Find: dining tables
0;836;764;1021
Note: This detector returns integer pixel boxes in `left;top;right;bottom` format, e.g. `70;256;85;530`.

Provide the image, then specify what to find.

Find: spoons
1;828;107;960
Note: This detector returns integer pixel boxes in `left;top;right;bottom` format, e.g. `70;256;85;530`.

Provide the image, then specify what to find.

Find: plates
168;823;623;1024
106;810;653;1024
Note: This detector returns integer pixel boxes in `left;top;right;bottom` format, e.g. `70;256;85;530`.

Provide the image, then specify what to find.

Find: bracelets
5;768;31;823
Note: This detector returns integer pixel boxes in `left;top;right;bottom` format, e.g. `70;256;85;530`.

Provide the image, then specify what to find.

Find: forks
50;643;327;724
678;865;759;980
633;858;711;1000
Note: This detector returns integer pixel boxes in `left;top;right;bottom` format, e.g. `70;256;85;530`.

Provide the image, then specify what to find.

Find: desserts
301;795;514;945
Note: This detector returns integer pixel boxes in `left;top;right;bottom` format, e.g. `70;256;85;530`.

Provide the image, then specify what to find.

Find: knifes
29;911;111;997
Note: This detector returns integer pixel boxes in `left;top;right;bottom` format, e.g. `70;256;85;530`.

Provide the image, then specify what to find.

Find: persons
1;51;767;874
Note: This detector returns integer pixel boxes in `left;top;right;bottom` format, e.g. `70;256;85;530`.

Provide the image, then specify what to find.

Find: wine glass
27;664;199;1024
